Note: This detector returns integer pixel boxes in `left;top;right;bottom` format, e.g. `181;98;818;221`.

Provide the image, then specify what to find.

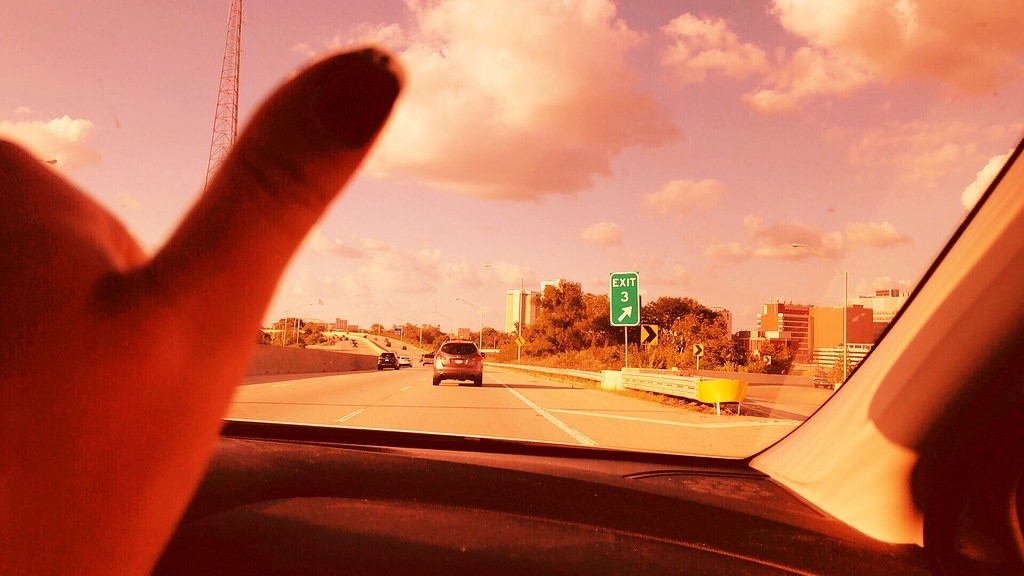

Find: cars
332;334;358;347
385;339;391;347
403;346;407;350
420;356;423;362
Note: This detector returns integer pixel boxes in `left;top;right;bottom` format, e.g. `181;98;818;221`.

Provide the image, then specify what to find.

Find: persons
0;48;407;576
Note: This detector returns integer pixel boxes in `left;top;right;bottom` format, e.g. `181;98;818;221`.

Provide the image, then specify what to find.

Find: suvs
433;340;485;386
422;353;435;366
377;352;400;370
399;357;413;367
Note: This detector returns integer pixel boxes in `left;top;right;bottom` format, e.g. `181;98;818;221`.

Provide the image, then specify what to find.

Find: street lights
456;298;483;355
283;303;313;347
484;264;523;365
433;311;452;341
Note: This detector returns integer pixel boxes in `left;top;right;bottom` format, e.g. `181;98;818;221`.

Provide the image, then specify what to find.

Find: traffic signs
610;273;641;327
